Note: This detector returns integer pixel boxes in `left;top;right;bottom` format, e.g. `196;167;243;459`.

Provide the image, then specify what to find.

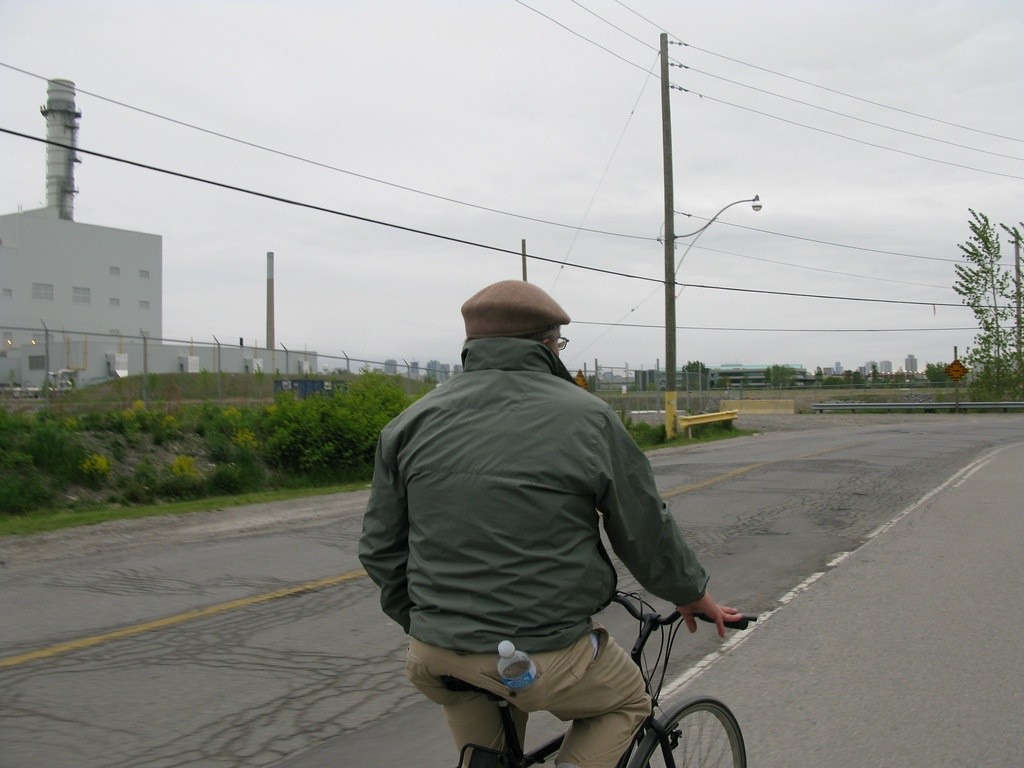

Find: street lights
658;195;763;442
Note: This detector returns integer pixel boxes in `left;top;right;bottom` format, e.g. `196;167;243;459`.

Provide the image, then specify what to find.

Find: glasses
558;335;569;351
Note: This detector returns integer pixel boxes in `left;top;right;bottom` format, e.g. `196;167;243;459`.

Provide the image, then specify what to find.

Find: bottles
498;640;539;691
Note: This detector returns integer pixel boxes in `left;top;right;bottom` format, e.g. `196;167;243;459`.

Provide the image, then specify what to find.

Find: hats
461;280;571;339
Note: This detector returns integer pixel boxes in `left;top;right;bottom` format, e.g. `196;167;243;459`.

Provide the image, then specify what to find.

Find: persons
358;281;741;768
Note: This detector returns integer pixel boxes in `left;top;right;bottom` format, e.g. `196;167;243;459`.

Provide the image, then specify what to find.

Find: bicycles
441;588;758;768
